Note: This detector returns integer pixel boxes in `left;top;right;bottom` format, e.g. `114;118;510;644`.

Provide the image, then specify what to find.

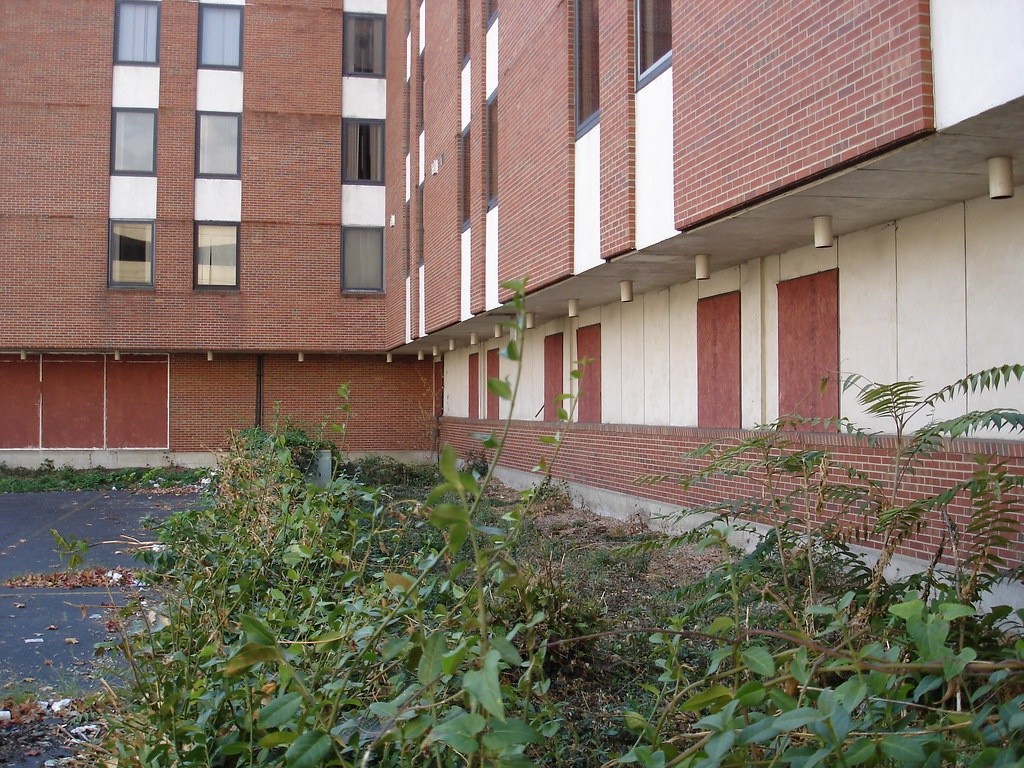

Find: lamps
568;299;580;318
695;254;712;281
298;352;304;362
386;352;392;363
525;312;534;330
20;350;28;360
813;216;834;249
449;339;456;351
619;280;634;303
432;345;438;356
418;350;425;361
207;351;214;361
494;322;503;339
114;351;121;361
986;155;1014;201
470;333;478;345
